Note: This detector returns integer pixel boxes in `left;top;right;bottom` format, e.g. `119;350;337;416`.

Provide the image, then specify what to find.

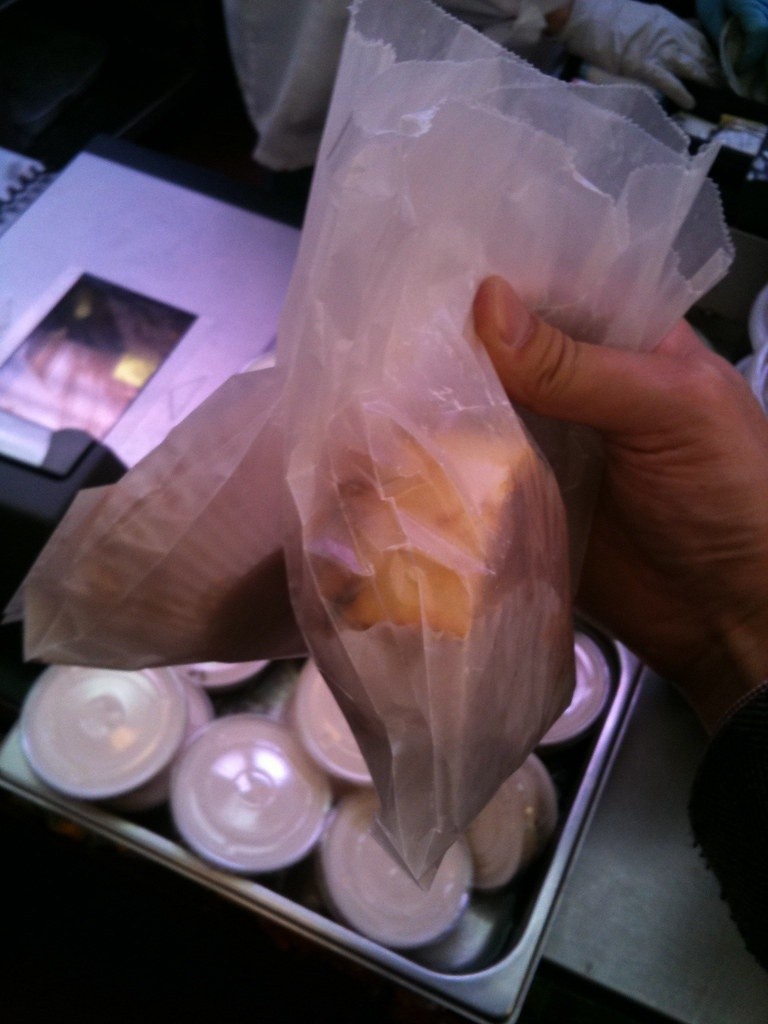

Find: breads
50;386;574;819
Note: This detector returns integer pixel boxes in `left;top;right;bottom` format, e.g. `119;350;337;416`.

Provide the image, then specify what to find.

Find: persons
473;276;768;974
221;0;767;233
